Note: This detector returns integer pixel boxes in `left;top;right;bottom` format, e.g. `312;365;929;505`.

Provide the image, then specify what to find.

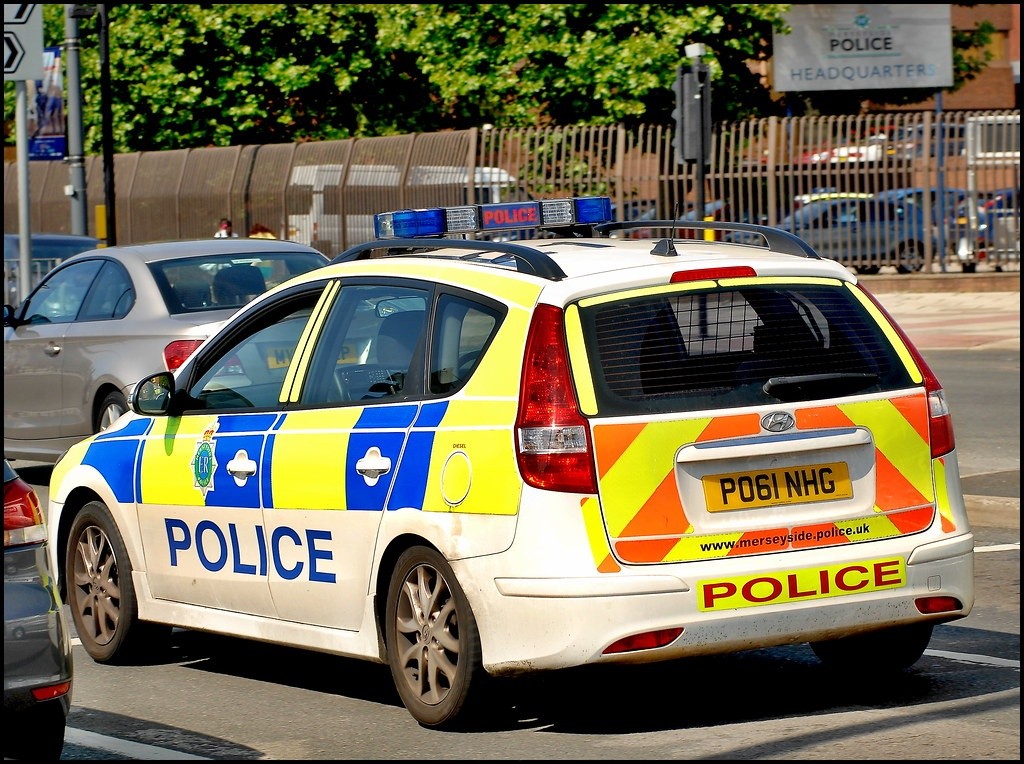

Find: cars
944;188;1021;273
5;237;416;464
732;195;937;274
842;187;969;224
3;454;73;763
611;199;730;243
48;196;975;730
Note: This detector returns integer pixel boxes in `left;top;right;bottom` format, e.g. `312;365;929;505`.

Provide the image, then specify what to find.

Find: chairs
213;264;266;307
375;311;428;378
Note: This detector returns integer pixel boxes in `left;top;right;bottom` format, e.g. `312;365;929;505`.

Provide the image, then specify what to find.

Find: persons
209;267;240;306
248;224;279;283
214;218;238;238
